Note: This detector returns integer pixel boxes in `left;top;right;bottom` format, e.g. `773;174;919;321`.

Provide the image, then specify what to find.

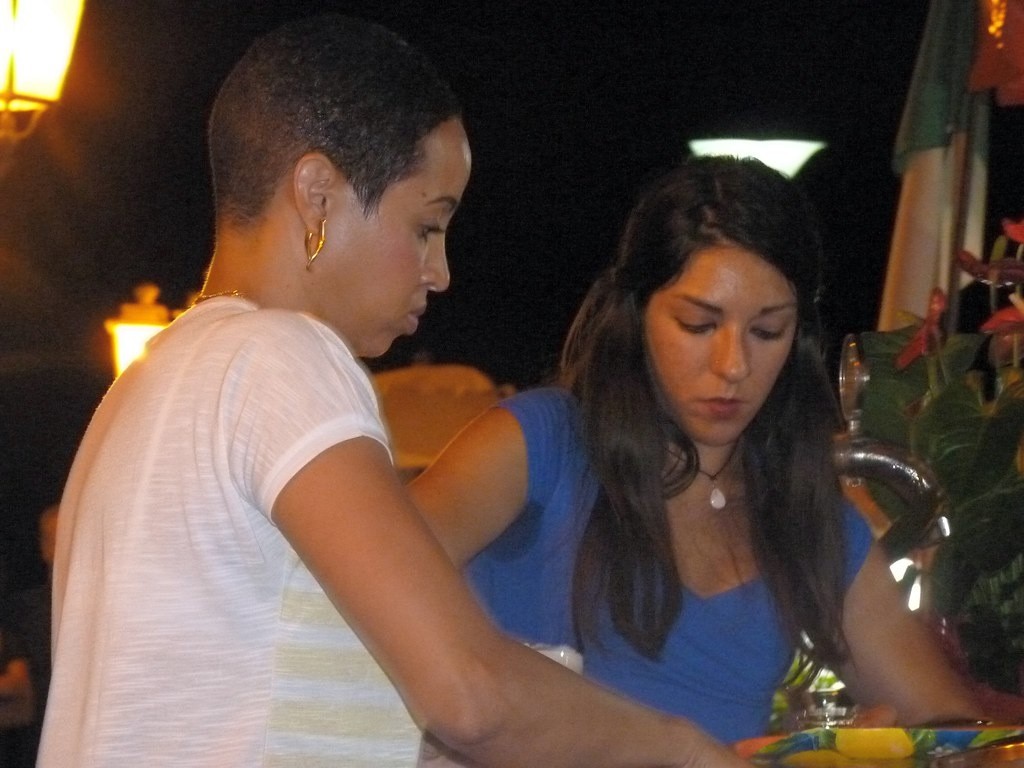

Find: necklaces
200;290;238;299
666;447;735;509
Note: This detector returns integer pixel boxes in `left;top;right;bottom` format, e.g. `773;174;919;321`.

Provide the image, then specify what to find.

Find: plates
728;725;1024;767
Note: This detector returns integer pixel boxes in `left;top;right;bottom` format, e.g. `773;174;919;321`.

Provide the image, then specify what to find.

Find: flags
879;0;984;334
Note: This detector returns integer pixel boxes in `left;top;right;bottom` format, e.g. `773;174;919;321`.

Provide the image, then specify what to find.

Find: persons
33;12;754;768
407;155;995;724
